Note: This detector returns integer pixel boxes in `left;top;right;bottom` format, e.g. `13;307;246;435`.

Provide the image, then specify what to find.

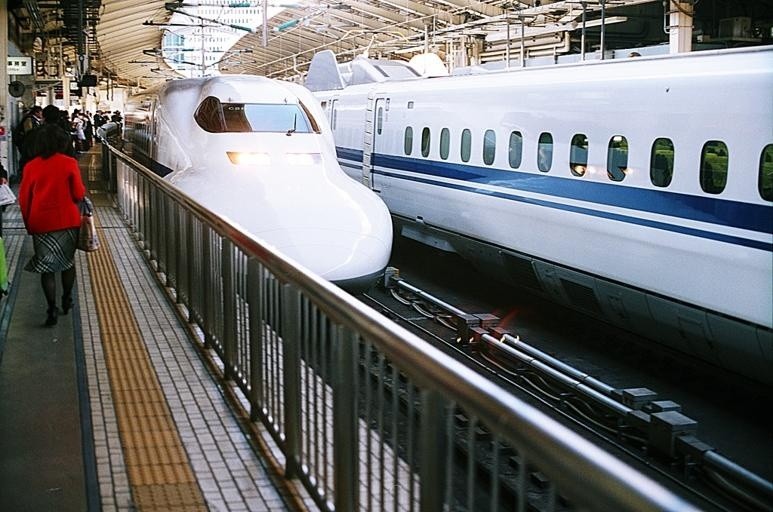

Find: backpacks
14;116;33;149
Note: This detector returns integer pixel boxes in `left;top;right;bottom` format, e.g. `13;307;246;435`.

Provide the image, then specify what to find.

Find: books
0;184;17;207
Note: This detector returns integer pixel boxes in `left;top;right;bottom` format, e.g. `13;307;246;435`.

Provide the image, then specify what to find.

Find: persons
12;104;123;185
15;122;86;329
0;160;10;301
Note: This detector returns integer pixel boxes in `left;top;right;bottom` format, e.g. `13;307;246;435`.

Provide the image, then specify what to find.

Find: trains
123;72;396;295
288;43;773;394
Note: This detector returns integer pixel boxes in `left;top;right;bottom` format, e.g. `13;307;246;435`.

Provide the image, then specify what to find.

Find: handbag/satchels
78;194;101;252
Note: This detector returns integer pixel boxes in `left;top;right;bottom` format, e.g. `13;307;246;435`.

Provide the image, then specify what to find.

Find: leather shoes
46;309;58;328
62;297;75;314
